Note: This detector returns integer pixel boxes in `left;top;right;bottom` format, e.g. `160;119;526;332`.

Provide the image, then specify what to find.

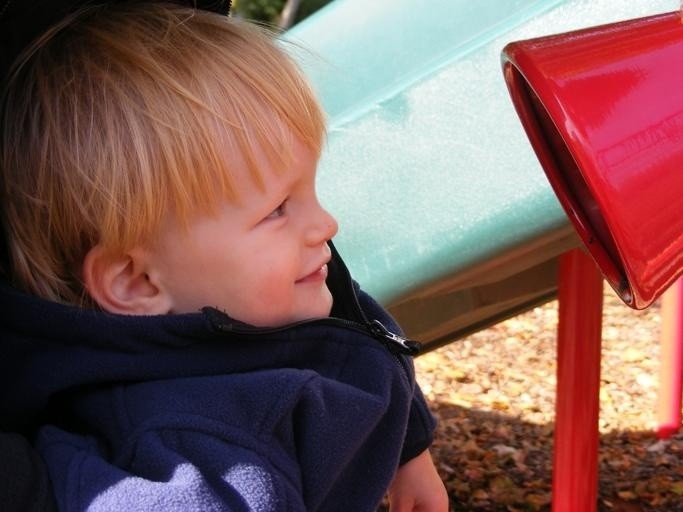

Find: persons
1;1;450;511
